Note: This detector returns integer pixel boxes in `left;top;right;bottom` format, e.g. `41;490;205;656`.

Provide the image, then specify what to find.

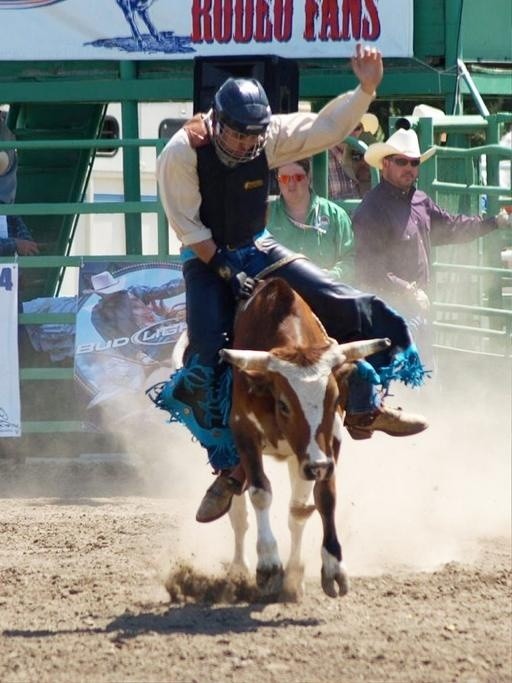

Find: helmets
211;74;278;135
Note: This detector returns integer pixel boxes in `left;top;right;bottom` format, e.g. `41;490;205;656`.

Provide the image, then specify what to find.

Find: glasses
387;155;423;168
276;172;306;184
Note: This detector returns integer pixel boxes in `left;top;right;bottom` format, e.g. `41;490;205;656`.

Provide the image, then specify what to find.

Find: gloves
404;280;431;313
206;251;256;298
494;205;512;230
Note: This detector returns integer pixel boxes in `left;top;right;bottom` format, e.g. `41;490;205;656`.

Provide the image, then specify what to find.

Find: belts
213;235;252;253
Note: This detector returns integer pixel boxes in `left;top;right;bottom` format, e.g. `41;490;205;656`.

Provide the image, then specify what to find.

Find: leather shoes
194;467;249;526
342;406;429;440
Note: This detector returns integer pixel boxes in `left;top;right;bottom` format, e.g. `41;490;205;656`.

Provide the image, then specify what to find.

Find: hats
360;111;379;136
365;126;440;177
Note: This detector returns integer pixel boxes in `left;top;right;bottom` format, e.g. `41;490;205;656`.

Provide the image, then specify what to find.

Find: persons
85;267;188;381
151;48;430;525
260;103;510;317
0;106;40;289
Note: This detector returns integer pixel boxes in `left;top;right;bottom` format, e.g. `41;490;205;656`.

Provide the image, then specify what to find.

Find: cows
187;272;393;600
